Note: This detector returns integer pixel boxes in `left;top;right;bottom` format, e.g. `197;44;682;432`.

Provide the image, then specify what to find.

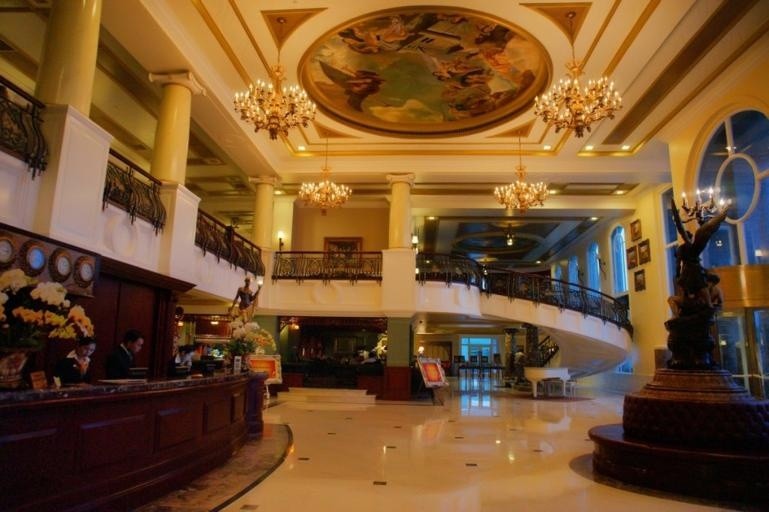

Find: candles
682;187;734;210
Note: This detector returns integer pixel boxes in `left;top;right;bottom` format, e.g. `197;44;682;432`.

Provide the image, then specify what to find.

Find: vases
232;352;250;371
0;348;33;388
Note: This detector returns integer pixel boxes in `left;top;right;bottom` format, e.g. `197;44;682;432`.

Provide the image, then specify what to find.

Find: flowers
227;318;276;353
0;269;95;350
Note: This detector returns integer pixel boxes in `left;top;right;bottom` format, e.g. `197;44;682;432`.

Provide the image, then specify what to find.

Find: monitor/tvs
126;367;150;379
200;364;216;373
167;366;188;377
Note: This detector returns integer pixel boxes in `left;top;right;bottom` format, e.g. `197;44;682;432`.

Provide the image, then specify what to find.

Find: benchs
543;380;576;397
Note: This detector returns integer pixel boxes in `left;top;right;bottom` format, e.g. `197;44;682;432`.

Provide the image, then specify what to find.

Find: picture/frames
625;219;650;292
324;237;363;268
416;357;450;388
245;355;283;385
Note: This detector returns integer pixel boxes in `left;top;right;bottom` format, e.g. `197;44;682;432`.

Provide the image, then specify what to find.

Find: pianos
524;368;571;398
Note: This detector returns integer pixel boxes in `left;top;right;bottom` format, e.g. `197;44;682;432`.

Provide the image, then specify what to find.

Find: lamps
277;231;284;250
493;131;549;214
298;133;353;216
534;11;623;139
411;236;418;249
505;222;516;246
232;18;317;139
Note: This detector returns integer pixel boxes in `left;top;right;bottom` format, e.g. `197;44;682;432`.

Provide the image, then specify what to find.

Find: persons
358;351;378;364
48;336;97;388
228;277;262;320
103;329;145;380
163;343;195;378
663;230;725;336
